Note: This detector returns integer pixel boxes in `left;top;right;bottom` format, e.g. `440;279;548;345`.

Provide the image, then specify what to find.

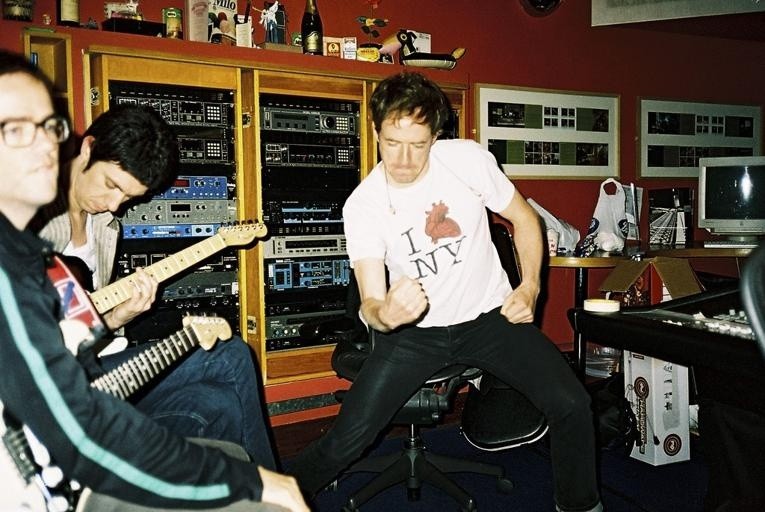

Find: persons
286;70;608;512
1;45;312;512
31;102;275;472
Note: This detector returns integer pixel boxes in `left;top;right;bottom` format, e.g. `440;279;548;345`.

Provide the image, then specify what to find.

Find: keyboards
703;240;760;249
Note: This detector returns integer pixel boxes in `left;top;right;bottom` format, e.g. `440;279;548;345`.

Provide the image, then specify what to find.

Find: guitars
0;310;233;512
47;219;268;357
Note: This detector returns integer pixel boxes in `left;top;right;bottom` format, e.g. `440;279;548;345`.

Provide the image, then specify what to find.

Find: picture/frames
474;83;621;181
635;97;764;180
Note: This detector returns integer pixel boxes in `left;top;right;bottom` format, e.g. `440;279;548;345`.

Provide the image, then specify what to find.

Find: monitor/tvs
697;155;765;240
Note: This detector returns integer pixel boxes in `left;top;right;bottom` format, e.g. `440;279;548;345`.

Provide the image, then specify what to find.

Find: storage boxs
624;350;691;466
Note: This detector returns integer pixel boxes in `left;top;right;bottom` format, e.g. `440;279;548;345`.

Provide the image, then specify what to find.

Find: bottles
299;0;325;57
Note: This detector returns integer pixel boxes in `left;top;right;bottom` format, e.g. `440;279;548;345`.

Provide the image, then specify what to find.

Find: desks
547;258;620;381
638;243;753;258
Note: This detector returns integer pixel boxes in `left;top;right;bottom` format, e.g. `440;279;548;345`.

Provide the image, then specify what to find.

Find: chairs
333;225;521;512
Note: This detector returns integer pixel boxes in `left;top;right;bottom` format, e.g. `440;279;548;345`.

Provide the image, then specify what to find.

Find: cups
545;228;560;257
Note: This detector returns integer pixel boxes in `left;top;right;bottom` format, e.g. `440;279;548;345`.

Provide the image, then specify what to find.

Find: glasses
0;113;70;147
